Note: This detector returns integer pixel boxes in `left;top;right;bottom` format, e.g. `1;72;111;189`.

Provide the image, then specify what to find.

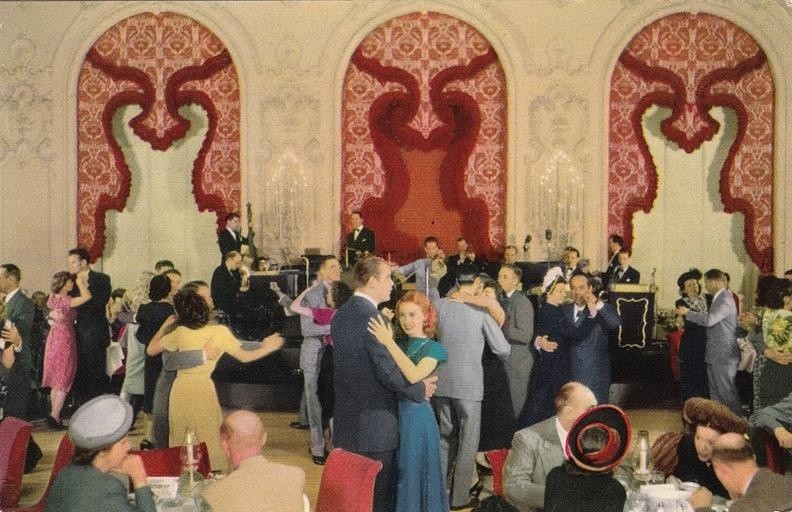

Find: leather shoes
450;498;479;511
289;422;309;429
47;414;64;429
309;446;330;465
23;452;42;474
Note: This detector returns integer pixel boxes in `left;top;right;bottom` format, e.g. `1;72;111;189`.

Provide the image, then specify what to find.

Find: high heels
470;480;483;498
141;439;154;451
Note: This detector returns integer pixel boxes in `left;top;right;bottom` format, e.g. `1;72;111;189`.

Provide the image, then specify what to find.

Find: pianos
482;260;587;293
237;269;306;299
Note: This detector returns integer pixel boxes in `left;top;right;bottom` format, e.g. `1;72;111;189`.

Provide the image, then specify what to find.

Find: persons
673;268;790;452
0;249;285;470
664;418;790;511
203;410;305;511
45;394;157;511
346;211;375;263
270;237;641;512
216;213;256;256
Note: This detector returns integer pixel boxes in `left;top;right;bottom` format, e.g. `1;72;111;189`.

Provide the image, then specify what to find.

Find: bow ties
353;228;359;232
617;267;624;273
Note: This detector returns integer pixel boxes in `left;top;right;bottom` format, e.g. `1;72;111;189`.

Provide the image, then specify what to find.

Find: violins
430;255;447;278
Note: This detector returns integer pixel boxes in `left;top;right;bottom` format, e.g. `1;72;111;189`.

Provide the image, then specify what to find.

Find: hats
564;404;632;473
680;396;750;437
68;394;134;452
678;270;699;287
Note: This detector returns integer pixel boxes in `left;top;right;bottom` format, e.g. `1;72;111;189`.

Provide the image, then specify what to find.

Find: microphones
545;229;552;240
613;264;625;287
651;268;656;277
523;235;532;252
